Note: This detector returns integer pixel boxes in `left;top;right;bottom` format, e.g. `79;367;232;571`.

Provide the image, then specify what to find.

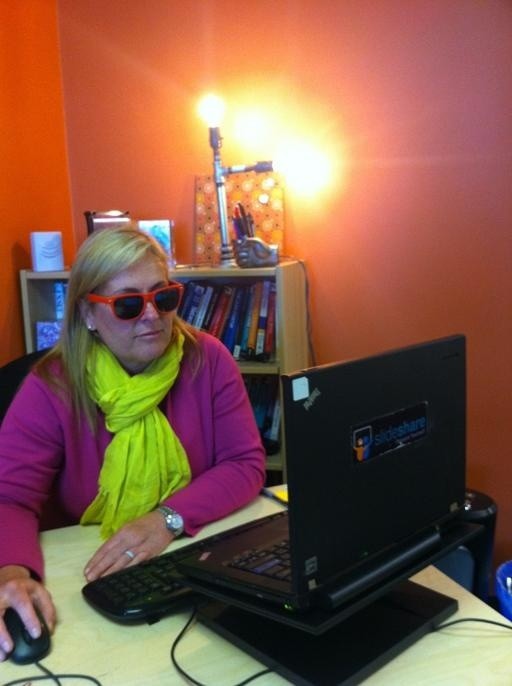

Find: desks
0;495;512;686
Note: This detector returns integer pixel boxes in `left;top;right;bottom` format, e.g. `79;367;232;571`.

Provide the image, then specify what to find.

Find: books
184;276;285;461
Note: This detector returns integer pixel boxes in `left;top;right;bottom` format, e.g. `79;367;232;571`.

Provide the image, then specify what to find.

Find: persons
1;227;266;666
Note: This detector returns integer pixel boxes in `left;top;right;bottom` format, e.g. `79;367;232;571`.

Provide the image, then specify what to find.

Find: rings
125;549;137;558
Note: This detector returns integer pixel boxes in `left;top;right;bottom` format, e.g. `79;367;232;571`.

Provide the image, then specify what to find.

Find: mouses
3;606;50;664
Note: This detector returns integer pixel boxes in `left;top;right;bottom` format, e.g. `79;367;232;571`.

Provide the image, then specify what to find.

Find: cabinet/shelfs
20;260;310;485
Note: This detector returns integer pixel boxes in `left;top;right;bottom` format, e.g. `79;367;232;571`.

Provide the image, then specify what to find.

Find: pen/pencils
232;202;254;239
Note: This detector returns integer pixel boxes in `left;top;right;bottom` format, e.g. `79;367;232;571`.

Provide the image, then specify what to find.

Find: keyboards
81;508;288;624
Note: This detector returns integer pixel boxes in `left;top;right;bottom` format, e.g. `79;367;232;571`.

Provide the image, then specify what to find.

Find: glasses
81;280;184;322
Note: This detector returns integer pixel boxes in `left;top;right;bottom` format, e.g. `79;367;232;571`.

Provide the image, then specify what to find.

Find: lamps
197;93;327;268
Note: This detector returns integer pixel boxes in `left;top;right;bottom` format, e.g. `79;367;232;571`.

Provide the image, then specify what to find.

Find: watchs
155;503;185;538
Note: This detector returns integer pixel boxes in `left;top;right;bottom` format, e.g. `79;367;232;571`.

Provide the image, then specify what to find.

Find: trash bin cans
496;559;512;622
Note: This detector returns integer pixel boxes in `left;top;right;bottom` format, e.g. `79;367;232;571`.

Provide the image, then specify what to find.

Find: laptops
177;332;469;618
